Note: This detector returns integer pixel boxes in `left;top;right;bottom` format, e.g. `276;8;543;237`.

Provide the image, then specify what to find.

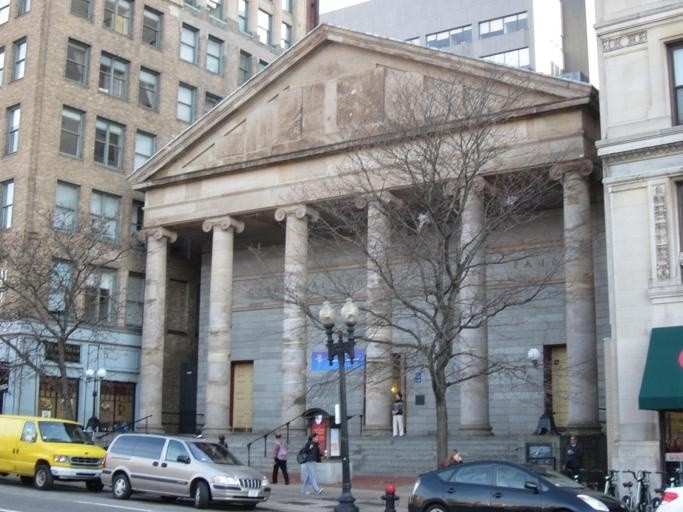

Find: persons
561;434;584;479
389;391;406;439
443;448;464;468
269;432;289;485
215;433;228;461
297;432;326;496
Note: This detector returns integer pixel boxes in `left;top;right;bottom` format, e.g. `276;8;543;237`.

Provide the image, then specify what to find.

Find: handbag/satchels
297;448;308;464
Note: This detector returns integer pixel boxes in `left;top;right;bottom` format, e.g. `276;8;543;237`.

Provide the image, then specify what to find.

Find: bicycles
561;468;683;512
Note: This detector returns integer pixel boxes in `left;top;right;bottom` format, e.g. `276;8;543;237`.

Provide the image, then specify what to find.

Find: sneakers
270;481;277;484
392;433;405;437
317;488;324;496
285;481;290;485
301;490;311;495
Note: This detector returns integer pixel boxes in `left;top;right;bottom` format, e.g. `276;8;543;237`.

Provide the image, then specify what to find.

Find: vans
0;414;107;492
100;433;272;509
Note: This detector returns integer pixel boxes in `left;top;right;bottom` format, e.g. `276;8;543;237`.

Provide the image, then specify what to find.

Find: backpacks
277;441;289;460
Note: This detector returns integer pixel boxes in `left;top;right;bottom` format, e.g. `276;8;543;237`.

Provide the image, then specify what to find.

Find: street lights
85;368;107;432
319;297;361;512
528;348;559;436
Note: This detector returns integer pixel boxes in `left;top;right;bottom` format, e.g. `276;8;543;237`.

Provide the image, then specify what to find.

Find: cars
408;459;630;512
